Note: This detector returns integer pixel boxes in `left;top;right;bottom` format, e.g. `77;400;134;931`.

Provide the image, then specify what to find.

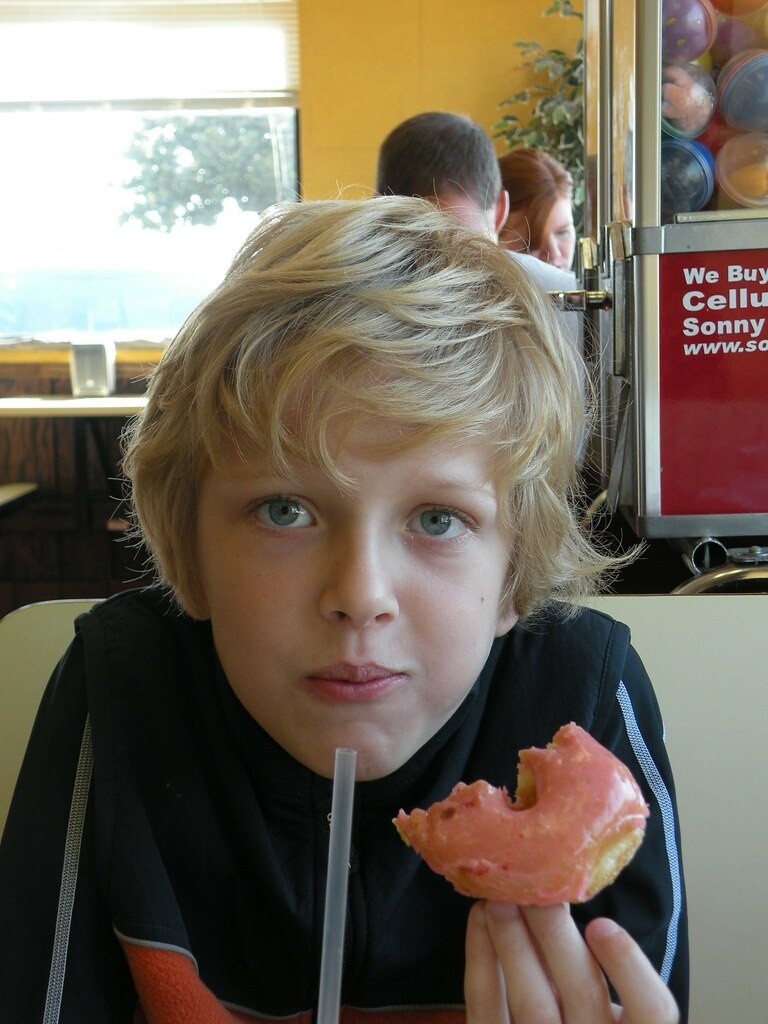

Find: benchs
0;592;768;1024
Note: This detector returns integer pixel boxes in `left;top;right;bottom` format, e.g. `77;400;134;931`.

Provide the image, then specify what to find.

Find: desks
0;394;154;599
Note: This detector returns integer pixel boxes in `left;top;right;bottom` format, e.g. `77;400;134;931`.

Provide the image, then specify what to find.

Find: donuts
392;719;650;909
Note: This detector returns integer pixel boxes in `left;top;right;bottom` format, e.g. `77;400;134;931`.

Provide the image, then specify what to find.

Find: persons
497;146;577;272
373;111;509;246
0;196;691;1024
